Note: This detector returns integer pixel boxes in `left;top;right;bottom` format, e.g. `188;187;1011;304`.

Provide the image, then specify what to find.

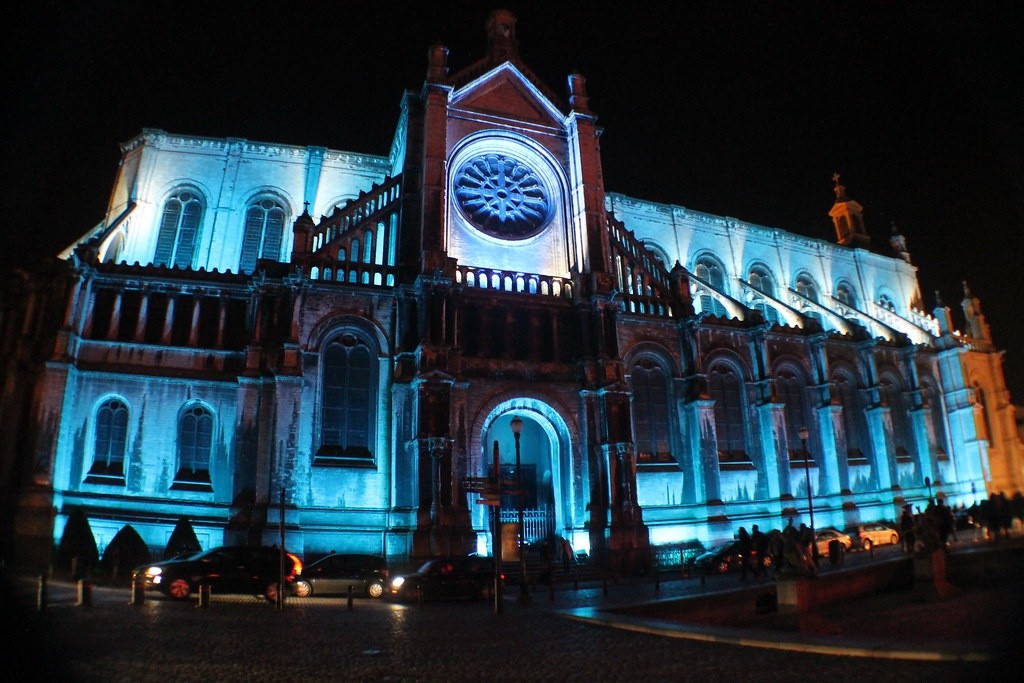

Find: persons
739;519;812;581
900;499;959;553
986;492;1024;538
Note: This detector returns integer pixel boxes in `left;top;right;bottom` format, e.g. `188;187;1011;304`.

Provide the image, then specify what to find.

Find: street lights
798;426;818;565
511;415;533;608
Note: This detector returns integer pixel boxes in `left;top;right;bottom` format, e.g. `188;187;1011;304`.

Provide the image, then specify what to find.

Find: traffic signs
461;476;524;507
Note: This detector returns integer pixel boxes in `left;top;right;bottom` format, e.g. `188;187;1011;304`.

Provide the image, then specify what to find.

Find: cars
844;523;899;551
296;553;388;599
131;541;302;601
694;528;852;574
945;513;993;544
390;558;506;600
